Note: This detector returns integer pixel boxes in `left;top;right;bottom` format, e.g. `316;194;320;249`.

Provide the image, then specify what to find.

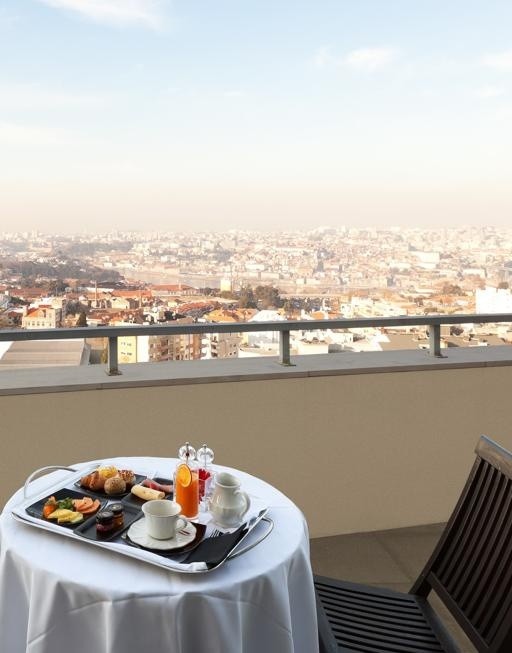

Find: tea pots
209;473;251;529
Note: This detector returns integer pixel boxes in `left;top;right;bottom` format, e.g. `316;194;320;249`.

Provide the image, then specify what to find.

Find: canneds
107;503;125;529
96;511;114;535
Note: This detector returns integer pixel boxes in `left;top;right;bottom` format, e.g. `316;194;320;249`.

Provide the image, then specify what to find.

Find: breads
80;466;136;490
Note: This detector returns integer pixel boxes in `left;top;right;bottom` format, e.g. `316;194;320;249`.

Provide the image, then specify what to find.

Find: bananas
131;485;165;500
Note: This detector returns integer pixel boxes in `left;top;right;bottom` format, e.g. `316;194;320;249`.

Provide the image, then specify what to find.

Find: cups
140;500;188;543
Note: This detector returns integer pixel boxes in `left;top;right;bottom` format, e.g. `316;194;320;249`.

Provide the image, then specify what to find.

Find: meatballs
104;477;126;495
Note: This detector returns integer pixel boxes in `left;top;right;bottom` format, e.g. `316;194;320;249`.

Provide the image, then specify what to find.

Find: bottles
196;443;215;503
175;441;199;518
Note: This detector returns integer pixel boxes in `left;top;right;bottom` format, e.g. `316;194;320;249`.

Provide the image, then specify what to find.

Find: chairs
315;429;511;652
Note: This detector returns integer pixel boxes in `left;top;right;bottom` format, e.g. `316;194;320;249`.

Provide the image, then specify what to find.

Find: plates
74;502;143;542
119;520;209;556
122;476;174;507
74;468;147;500
24;487;107;527
127;518;196;552
